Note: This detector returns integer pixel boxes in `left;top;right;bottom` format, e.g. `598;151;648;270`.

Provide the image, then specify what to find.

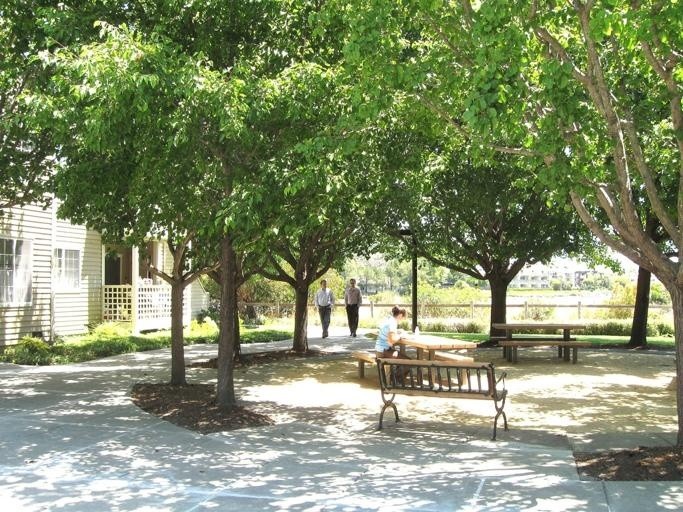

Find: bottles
415;325;419;337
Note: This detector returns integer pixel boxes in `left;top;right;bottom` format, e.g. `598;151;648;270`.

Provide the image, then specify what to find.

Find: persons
374;304;412;382
313;280;334;339
343;278;361;338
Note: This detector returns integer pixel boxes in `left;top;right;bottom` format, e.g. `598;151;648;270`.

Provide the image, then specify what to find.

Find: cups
402;331;407;336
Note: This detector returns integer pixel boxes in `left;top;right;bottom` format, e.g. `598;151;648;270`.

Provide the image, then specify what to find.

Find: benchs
423;350;474;385
490;336;576;359
374;357;509;442
498;340;592;363
351;350;391;386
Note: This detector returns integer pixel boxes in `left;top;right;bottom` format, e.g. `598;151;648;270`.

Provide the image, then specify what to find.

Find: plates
400;335;410;338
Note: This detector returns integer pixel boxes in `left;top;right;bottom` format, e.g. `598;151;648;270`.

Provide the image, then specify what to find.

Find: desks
396;334;478;394
492;323;586;360
